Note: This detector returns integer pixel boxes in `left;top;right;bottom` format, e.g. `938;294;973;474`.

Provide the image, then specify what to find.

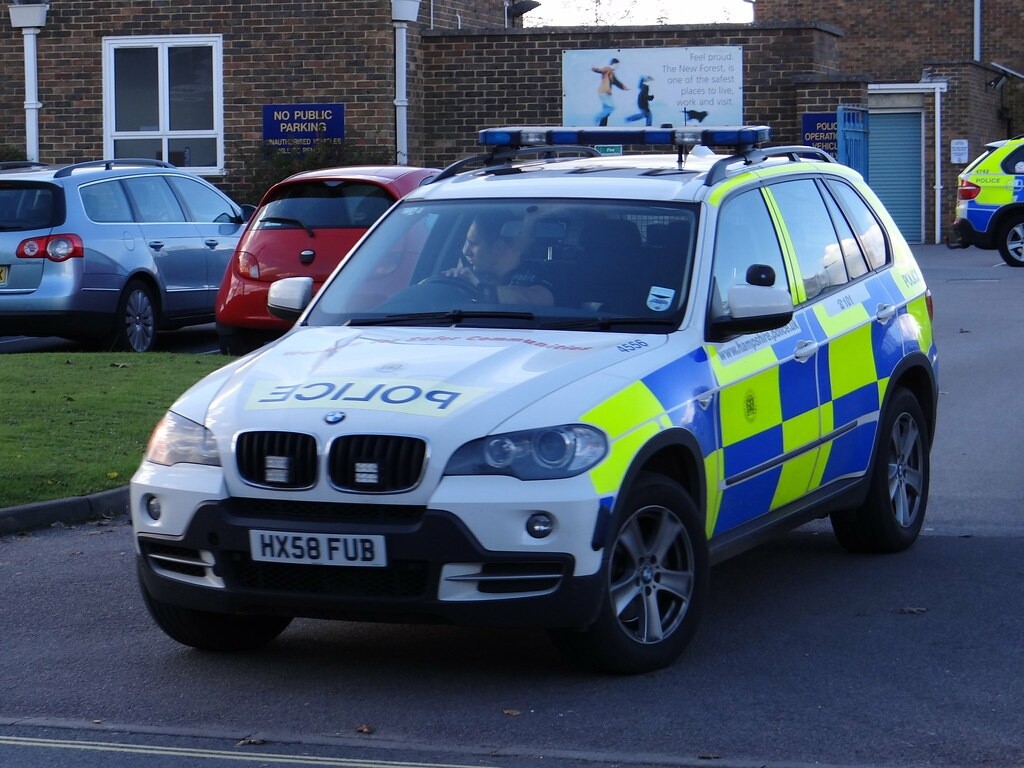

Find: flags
685;110;707;122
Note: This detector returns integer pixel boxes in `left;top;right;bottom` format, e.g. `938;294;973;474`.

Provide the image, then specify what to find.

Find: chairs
576;216;645;310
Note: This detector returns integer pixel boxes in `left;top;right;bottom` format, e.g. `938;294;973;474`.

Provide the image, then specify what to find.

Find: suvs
127;123;942;675
0;157;256;354
952;132;1024;268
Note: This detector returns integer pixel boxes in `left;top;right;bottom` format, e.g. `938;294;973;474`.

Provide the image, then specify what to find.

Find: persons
626;76;656;127
591;59;630;127
437;208;570;312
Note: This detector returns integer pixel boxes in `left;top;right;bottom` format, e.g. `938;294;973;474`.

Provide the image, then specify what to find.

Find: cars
213;166;457;359
